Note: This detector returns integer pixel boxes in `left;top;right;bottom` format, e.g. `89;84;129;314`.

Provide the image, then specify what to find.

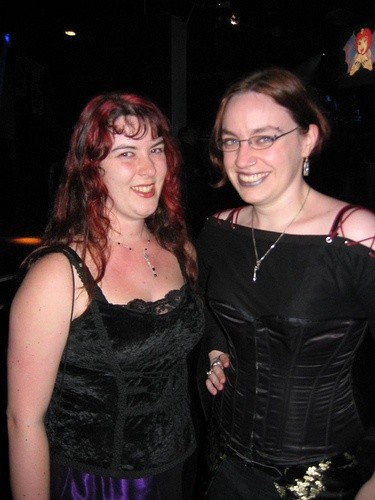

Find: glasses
216;126;298;152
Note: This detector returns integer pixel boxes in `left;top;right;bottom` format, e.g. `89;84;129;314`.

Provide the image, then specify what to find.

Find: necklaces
106;232;157;277
252;186;311;282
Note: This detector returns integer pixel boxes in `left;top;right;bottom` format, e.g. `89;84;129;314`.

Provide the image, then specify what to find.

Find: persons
7;94;230;500
192;66;375;500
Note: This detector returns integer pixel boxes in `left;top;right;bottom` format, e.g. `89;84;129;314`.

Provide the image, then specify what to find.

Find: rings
211;361;223;369
206;369;213;376
